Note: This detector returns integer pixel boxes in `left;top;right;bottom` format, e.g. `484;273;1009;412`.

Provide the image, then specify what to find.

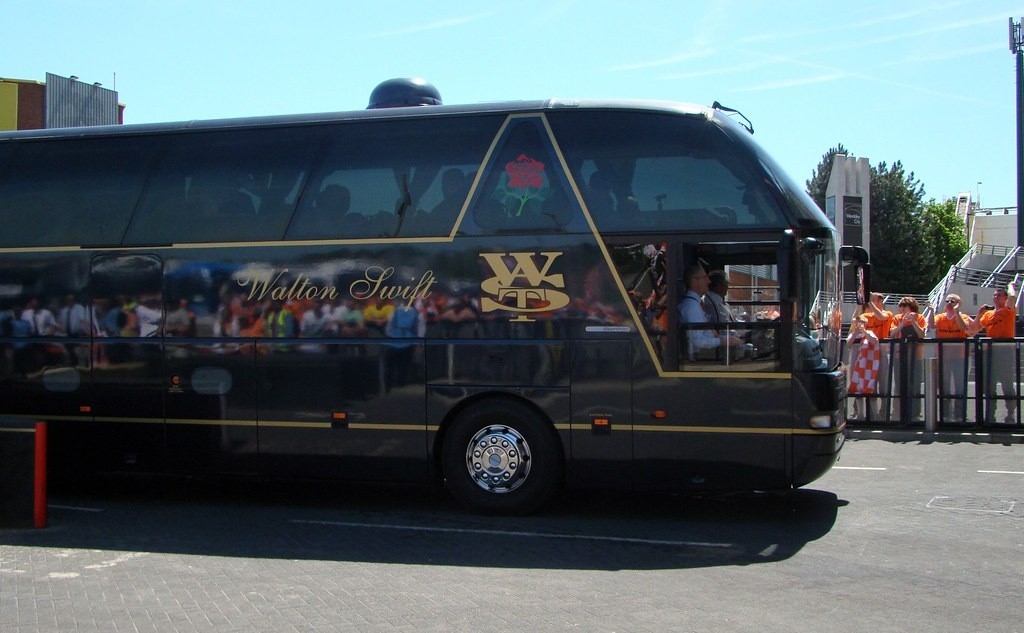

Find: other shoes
1001;416;1014;424
849;412;857;419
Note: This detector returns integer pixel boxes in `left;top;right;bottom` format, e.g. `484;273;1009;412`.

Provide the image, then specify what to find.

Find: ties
65;309;71;336
33;314;38;336
700;300;719;338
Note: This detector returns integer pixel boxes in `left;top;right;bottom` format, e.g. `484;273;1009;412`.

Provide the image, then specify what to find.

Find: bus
0;77;869;515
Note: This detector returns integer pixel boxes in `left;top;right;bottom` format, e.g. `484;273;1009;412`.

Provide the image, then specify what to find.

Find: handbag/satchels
847;337;879;394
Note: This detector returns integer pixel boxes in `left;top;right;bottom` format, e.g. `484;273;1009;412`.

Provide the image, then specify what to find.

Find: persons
682;258;756;366
140;148;641;247
13;244;676;377
761;305;780;319
845;281;1017;425
808;301;842;353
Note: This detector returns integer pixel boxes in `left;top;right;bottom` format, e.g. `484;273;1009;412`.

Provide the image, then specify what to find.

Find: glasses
898;303;907;308
692;275;708;281
945;300;955;304
